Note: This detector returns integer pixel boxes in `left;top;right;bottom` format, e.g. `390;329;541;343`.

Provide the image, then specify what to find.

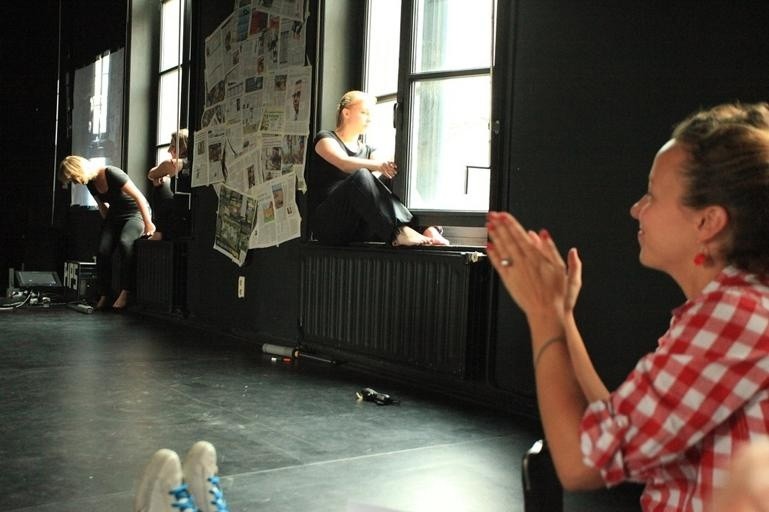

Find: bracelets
532;335;564;371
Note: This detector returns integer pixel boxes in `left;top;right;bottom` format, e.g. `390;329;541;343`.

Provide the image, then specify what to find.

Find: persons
59;156;160;310
147;129;191;244
484;101;769;512
290;80;303;119
305;88;451;247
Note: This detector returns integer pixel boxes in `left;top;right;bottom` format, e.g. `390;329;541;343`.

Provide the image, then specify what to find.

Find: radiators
298;246;486;381
109;239;185;314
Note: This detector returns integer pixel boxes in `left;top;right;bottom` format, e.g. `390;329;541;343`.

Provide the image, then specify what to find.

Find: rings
498;258;512;267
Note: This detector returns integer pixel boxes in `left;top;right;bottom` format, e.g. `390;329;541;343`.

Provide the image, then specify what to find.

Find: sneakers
136;440;229;512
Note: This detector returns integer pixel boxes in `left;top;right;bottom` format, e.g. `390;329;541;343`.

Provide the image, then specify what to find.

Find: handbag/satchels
522;438;563;511
